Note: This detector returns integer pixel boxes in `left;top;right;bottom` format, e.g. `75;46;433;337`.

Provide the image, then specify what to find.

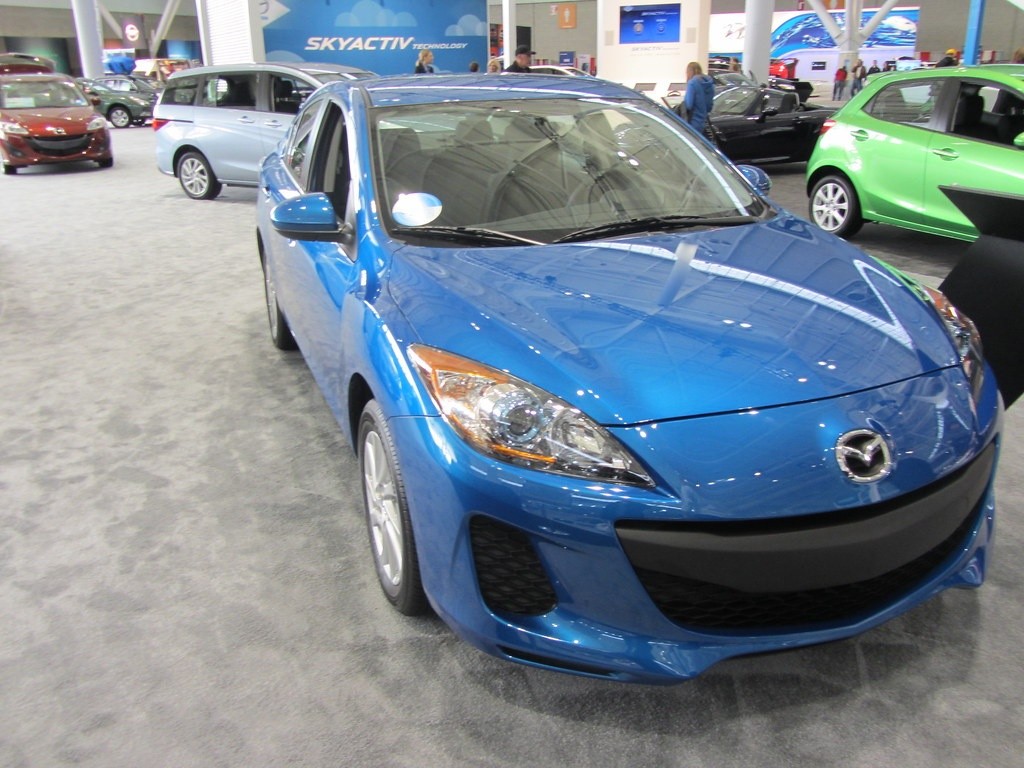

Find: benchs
881;90;930;118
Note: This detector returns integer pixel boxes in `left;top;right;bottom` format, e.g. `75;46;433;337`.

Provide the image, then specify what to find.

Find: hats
515;45;536;56
945;49;957;57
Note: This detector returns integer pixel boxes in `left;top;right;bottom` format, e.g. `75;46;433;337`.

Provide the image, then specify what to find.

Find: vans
131;58;191;85
152;63;461;201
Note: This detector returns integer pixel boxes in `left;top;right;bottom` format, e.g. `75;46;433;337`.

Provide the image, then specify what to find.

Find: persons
470;52;501;74
503;45;536;72
850;59;867;97
987;48;1024;115
684;62;714;134
935;49;959;67
832;66;847;101
770;60;787;78
868;60;897;74
415;50;434;73
729;56;740;73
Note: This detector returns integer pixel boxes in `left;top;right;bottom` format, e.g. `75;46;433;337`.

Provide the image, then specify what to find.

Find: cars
498;65;589;77
654;73;842;165
806;55;1024;241
0;52;113;176
255;71;1005;687
73;77;159;128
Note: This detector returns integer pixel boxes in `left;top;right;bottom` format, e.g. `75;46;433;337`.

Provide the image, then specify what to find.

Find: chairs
459;122;494;155
777;92;800;113
995;90;1024;145
276;80;299;115
373;129;421;180
956;96;985;136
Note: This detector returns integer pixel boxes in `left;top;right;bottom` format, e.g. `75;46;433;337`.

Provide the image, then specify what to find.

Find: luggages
707;113;740;162
